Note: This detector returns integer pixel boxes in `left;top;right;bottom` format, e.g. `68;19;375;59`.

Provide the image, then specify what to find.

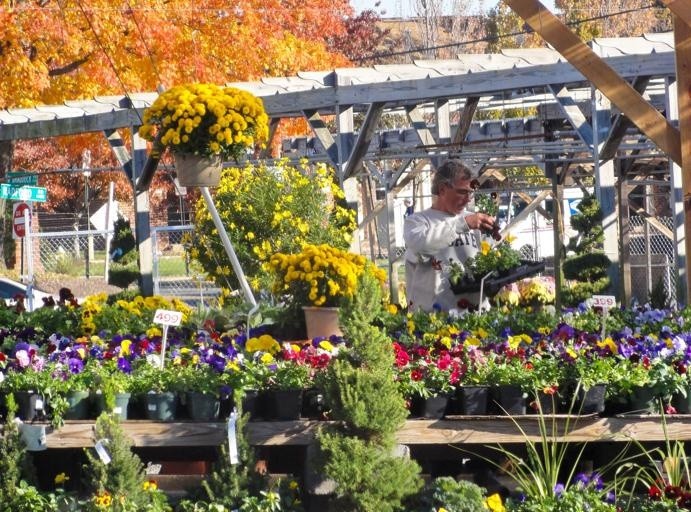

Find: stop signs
13;201;32;238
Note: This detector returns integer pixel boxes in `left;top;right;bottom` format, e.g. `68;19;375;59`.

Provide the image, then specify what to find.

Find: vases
175;147;218;186
454;257;540;305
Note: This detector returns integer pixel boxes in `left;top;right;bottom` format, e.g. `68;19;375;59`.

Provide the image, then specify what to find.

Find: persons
403;166;522;313
403;195;417;219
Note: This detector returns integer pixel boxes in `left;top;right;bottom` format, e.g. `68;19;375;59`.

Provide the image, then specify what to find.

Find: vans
475;186;595;261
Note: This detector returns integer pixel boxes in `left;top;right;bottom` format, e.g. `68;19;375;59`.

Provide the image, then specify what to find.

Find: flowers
14;303;691;423
142;84;268;146
273;244;384;300
191;161;354;275
0;414;691;512
443;236;519;276
